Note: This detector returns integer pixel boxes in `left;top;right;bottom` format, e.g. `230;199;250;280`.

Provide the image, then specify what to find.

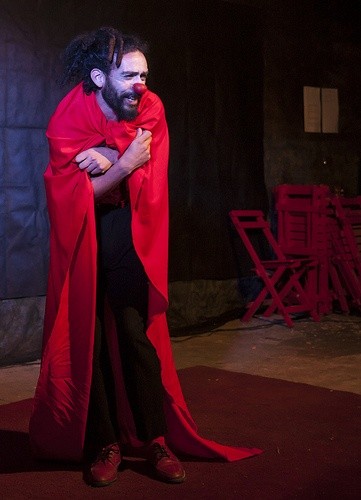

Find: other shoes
144;436;187;483
88;439;124;486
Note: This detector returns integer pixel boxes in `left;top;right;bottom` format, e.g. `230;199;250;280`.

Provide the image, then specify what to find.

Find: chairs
277;182;360;318
230;208;322;326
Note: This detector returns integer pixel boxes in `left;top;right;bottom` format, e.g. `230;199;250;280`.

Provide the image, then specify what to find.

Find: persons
29;25;270;489
